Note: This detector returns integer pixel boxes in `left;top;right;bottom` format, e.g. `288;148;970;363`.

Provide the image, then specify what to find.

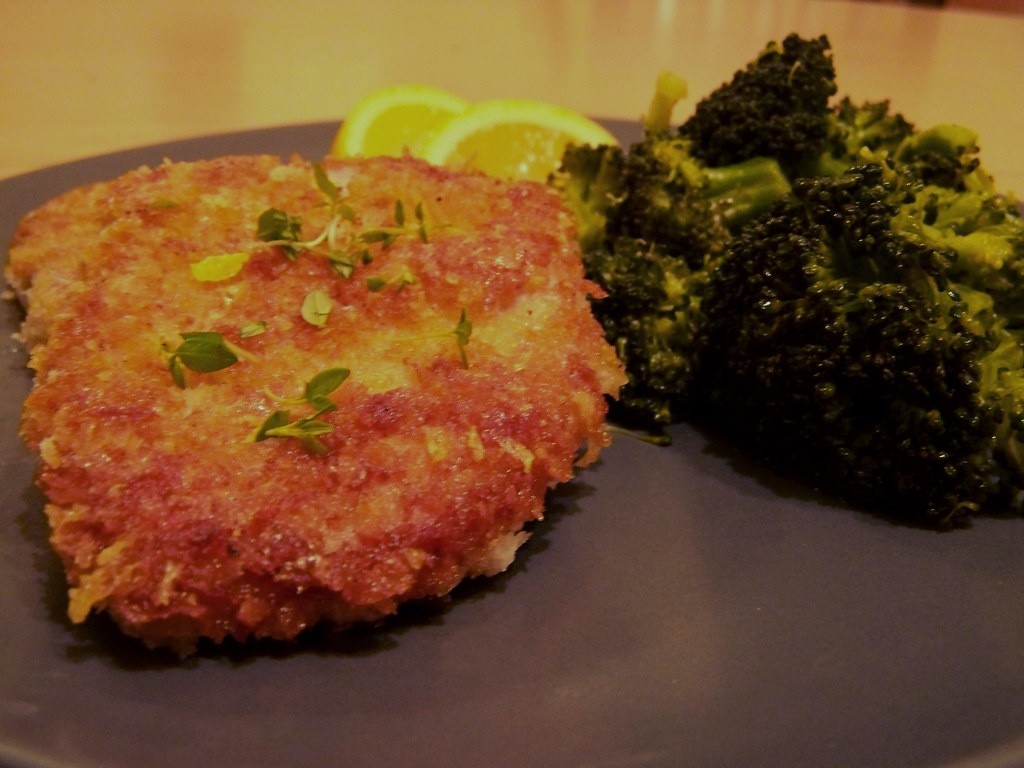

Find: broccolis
559;34;1024;529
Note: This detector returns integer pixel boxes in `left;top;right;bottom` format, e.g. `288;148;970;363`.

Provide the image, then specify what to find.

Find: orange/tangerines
330;86;628;203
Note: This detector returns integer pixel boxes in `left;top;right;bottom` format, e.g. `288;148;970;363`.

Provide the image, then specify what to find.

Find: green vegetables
138;166;472;454
